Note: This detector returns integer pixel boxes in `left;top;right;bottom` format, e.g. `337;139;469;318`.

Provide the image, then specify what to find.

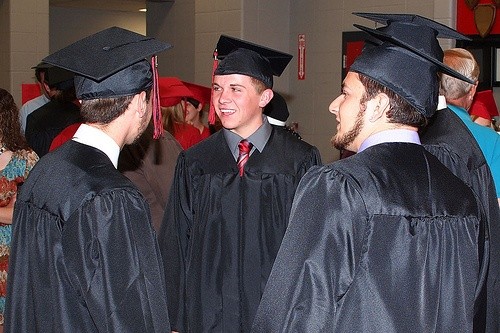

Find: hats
206;34;293;126
41;26;174;140
158;76;213;121
349;24;478;124
352;10;473;62
468;90;500;121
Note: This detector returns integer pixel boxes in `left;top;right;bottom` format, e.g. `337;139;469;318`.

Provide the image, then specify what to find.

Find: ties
236;140;251;176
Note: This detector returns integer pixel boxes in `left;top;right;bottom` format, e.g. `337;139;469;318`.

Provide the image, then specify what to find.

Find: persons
6;26;169;333
157;34;323;333
250;24;490;333
0;59;289;333
352;12;500;333
